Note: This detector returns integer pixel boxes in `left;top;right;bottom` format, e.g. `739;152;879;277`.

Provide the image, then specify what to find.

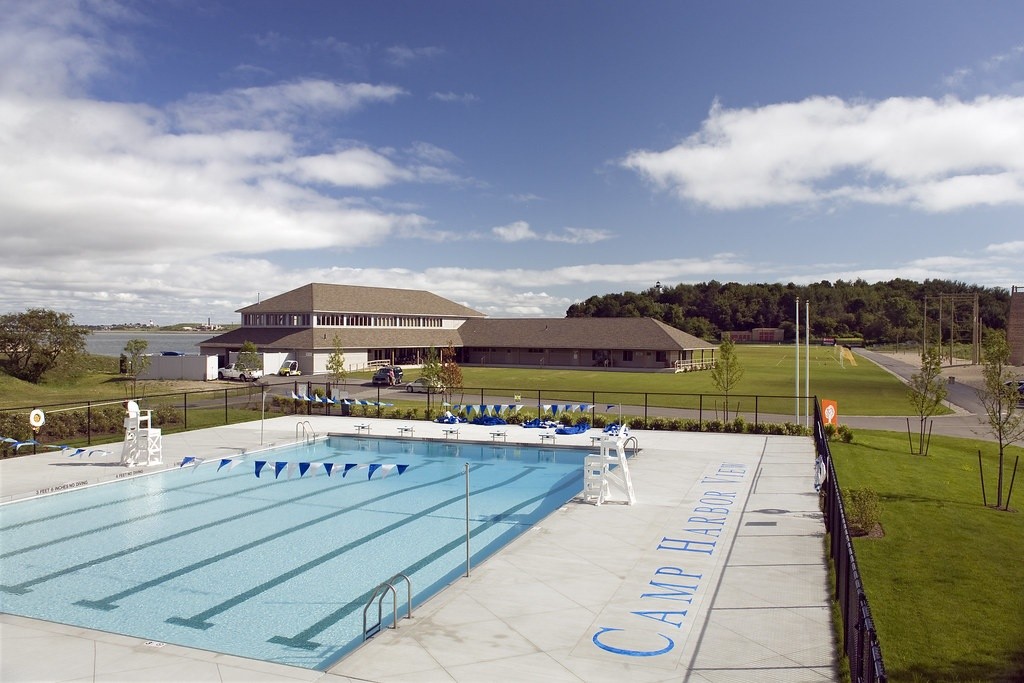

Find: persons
119;353;127;374
389;369;396;386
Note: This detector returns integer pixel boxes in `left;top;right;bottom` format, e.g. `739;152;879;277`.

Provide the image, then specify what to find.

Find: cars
372;367;403;386
161;350;186;357
998;380;1024;403
405;377;446;394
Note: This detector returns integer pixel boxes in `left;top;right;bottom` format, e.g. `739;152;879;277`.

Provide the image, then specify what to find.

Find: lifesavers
816;461;827;486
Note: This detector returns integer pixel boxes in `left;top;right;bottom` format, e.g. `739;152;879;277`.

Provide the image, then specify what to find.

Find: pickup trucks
218;363;264;383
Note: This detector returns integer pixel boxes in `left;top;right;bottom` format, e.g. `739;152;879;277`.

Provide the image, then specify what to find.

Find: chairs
127;400;163;465
582;424;638;506
